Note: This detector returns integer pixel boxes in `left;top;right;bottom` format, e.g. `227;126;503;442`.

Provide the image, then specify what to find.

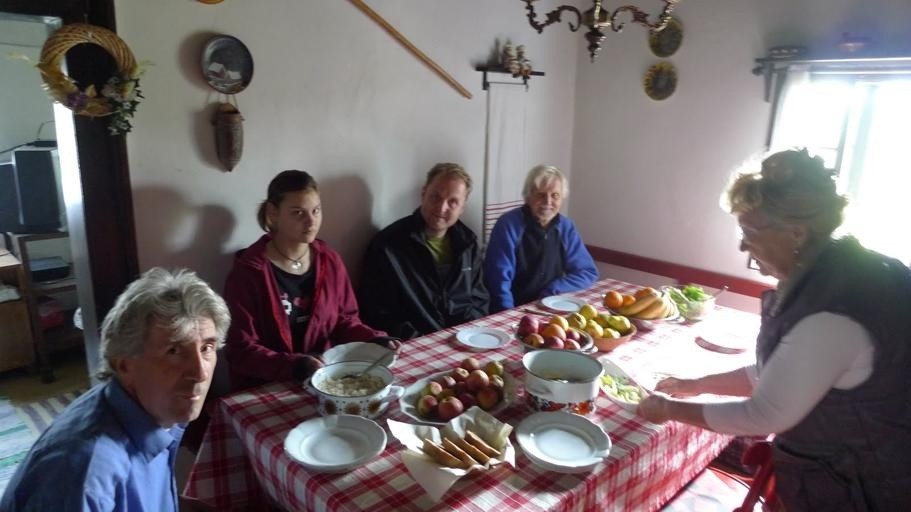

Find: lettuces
665;282;705;321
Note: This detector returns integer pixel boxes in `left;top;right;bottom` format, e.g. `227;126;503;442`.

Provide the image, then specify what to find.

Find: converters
35;140;56;147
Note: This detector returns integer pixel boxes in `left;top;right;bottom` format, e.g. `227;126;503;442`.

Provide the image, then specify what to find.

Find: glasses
735;223;776;240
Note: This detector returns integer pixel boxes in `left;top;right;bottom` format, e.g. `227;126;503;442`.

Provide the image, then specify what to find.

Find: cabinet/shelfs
8;231;89;383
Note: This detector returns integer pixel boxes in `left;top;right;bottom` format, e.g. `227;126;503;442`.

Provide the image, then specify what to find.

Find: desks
182;277;772;511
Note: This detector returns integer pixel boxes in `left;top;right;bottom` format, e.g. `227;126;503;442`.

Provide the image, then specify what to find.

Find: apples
415;358;506;422
516;313;581;351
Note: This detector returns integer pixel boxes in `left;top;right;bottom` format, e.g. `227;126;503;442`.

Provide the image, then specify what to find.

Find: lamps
525;0;674;64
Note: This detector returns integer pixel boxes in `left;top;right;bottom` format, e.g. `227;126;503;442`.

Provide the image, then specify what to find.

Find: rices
316;372;387;397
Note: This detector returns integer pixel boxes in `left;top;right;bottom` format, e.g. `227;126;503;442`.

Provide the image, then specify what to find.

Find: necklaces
269;240;313;271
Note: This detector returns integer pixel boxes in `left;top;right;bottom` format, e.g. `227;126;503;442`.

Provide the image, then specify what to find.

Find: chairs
483;200;527;257
659;440;775;512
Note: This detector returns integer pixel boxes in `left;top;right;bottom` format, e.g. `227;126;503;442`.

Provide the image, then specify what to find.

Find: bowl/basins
661;279;717;323
522;347;605;416
600;290;680;331
570;319;638;352
303;362;406;420
600;370;646;415
513;323;602;355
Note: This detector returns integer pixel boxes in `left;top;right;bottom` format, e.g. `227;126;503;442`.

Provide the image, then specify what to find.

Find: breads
423;430;502;469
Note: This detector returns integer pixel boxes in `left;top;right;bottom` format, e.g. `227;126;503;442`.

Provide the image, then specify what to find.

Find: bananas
617;290;677;319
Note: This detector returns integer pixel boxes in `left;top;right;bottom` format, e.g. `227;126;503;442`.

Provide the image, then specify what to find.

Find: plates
649;16;685;60
541;295;589;314
321;341;397;367
642;61;679;102
516;411;614;475
200;34;256;96
400;368;515;425
282;414;387;472
457;326;512;351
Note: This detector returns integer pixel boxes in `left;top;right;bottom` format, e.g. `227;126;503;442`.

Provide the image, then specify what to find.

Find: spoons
346;350;398;381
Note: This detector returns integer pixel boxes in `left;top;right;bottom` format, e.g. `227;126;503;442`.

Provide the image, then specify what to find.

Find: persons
1;262;235;510
482;164;603;317
357;160;494;344
633;144;910;510
221;168;406;389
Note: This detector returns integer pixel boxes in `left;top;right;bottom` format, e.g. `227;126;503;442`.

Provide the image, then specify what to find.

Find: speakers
12;141;69;234
0;162;17;233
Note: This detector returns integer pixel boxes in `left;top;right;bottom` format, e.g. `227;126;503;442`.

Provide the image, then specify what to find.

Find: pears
568;304;631;340
